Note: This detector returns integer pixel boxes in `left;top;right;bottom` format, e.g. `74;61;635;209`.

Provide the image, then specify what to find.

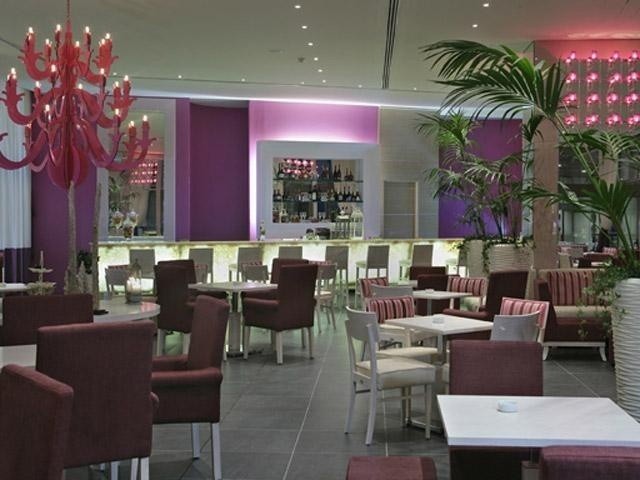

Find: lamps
0;1;151;192
124;161;159;187
278;157;318;175
561;48;638;129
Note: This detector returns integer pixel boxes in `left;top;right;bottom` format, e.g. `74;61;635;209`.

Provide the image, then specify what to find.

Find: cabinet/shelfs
272;178;364;223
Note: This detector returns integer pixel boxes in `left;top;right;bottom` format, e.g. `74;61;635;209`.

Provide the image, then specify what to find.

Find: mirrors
96;96;176;242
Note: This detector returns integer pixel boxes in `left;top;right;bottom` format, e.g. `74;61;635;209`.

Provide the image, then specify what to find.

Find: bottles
273;162;360;223
334;222;351;239
260;221;265;240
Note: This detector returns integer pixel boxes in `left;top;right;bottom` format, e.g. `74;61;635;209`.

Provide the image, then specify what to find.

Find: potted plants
417;38;640;424
453;188;492;277
409;105;549;273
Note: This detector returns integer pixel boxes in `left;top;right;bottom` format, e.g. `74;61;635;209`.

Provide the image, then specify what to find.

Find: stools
345;455;438;480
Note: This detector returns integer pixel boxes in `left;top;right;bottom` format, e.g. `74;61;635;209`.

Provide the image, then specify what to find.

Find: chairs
109;294;230;480
3;294;93;347
0;363;74;480
344;320;436;447
538;446;640;479
449;340;544;397
36;319;156;480
